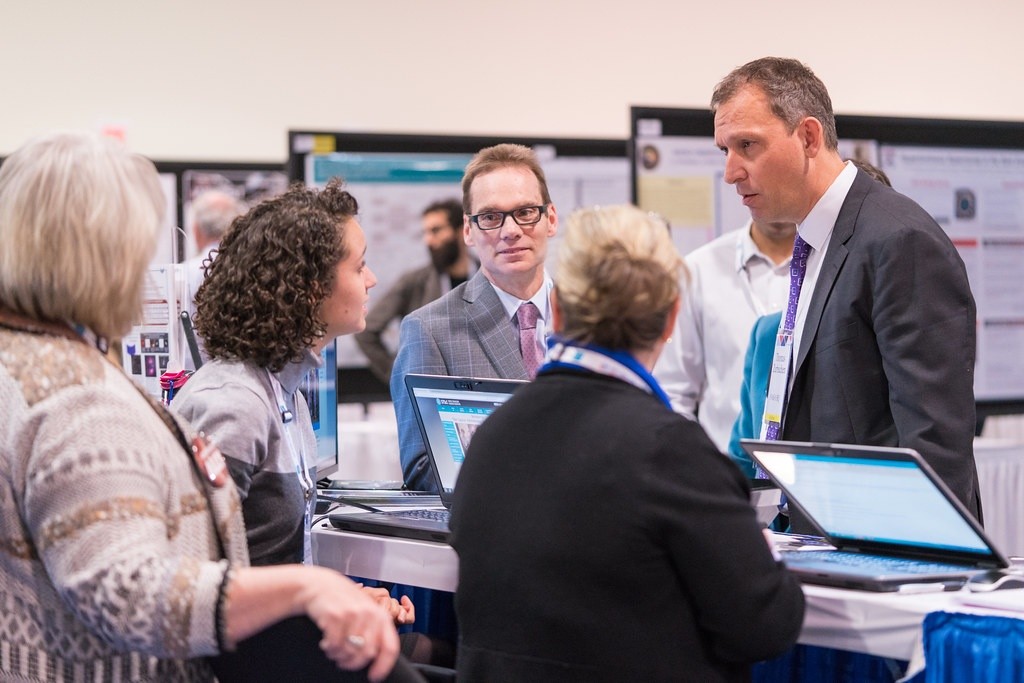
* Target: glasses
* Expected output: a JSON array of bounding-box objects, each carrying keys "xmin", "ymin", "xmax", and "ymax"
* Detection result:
[{"xmin": 462, "ymin": 202, "xmax": 548, "ymax": 232}]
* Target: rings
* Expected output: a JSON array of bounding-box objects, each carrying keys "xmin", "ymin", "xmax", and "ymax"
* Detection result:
[{"xmin": 346, "ymin": 635, "xmax": 363, "ymax": 645}]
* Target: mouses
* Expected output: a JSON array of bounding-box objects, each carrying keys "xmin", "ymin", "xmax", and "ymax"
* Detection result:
[{"xmin": 967, "ymin": 570, "xmax": 1023, "ymax": 593}]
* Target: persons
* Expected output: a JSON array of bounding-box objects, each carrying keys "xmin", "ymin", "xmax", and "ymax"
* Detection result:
[
  {"xmin": 165, "ymin": 177, "xmax": 437, "ymax": 664},
  {"xmin": 167, "ymin": 183, "xmax": 247, "ymax": 372},
  {"xmin": 711, "ymin": 57, "xmax": 985, "ymax": 533},
  {"xmin": 448, "ymin": 205, "xmax": 810, "ymax": 681},
  {"xmin": 355, "ymin": 195, "xmax": 478, "ymax": 382},
  {"xmin": 650, "ymin": 216, "xmax": 800, "ymax": 491},
  {"xmin": 0, "ymin": 135, "xmax": 400, "ymax": 683},
  {"xmin": 390, "ymin": 143, "xmax": 560, "ymax": 498}
]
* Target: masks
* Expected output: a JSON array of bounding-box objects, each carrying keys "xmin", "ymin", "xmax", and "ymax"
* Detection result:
[{"xmin": 429, "ymin": 237, "xmax": 460, "ymax": 275}]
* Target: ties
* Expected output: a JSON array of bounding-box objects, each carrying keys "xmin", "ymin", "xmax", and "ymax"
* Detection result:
[
  {"xmin": 514, "ymin": 300, "xmax": 546, "ymax": 384},
  {"xmin": 757, "ymin": 235, "xmax": 812, "ymax": 482}
]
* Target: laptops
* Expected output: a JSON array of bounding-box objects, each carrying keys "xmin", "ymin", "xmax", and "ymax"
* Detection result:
[
  {"xmin": 326, "ymin": 373, "xmax": 532, "ymax": 543},
  {"xmin": 739, "ymin": 438, "xmax": 1008, "ymax": 595}
]
